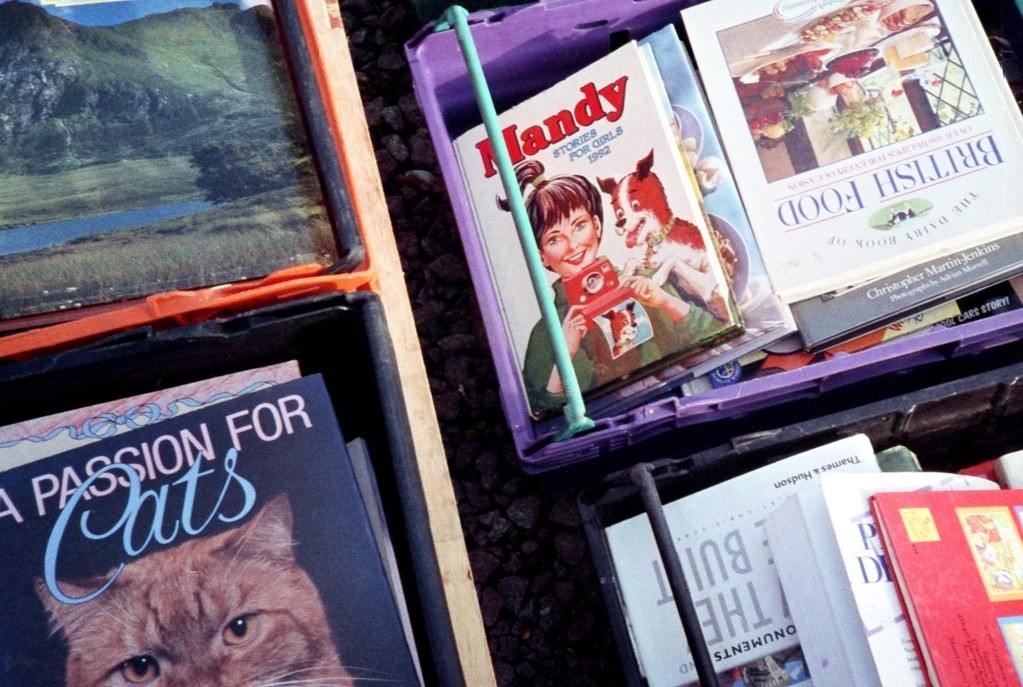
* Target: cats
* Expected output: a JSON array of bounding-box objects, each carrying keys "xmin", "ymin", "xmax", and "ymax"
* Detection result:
[{"xmin": 36, "ymin": 493, "xmax": 356, "ymax": 687}]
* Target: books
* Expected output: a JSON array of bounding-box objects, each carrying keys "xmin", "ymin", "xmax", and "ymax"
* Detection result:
[
  {"xmin": 451, "ymin": 0, "xmax": 1022, "ymax": 421},
  {"xmin": 602, "ymin": 432, "xmax": 1023, "ymax": 687},
  {"xmin": 0, "ymin": 0, "xmax": 340, "ymax": 333},
  {"xmin": 1, "ymin": 359, "xmax": 426, "ymax": 687}
]
